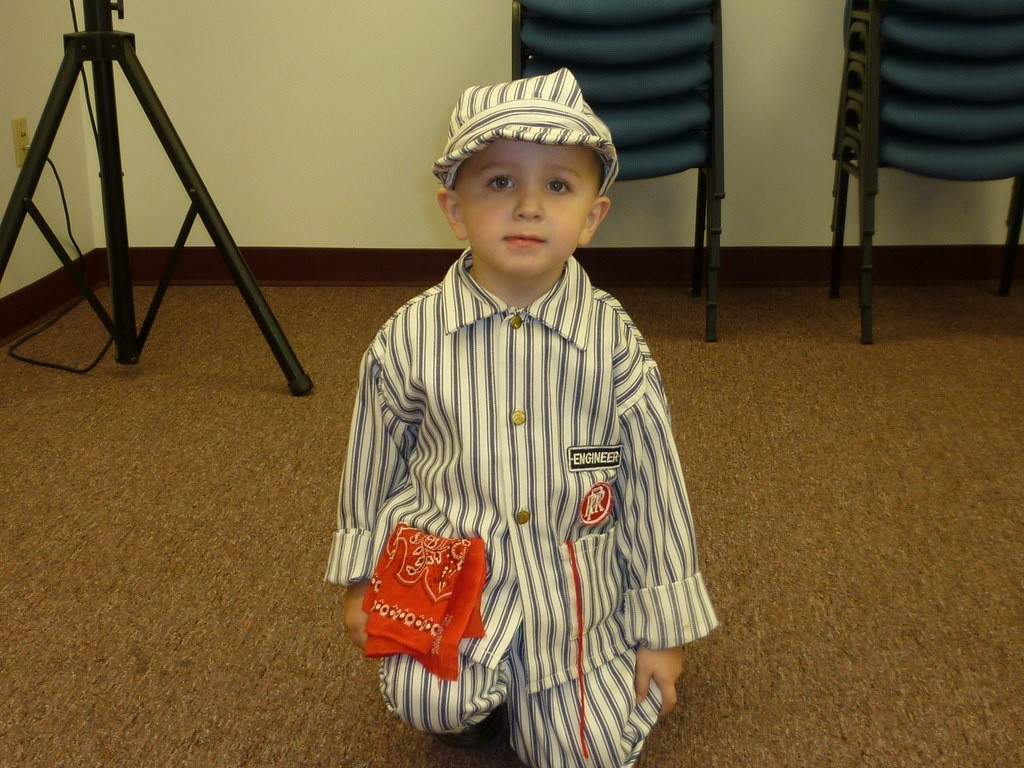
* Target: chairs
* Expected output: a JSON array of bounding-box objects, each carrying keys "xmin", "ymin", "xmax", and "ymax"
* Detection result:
[{"xmin": 515, "ymin": 1, "xmax": 1024, "ymax": 346}]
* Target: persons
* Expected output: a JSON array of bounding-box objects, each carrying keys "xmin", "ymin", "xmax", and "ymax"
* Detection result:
[{"xmin": 326, "ymin": 68, "xmax": 717, "ymax": 768}]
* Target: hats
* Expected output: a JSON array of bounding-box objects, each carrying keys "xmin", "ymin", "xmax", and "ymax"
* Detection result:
[{"xmin": 432, "ymin": 66, "xmax": 619, "ymax": 200}]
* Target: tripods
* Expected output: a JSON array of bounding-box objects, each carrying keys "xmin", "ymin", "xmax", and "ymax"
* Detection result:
[{"xmin": 0, "ymin": 0, "xmax": 314, "ymax": 397}]
